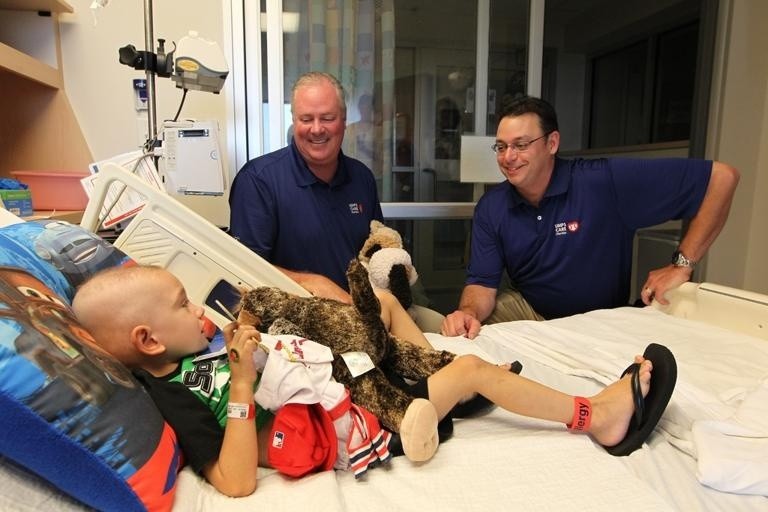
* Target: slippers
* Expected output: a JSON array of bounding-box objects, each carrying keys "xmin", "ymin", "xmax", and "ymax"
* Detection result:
[
  {"xmin": 452, "ymin": 360, "xmax": 522, "ymax": 419},
  {"xmin": 606, "ymin": 342, "xmax": 676, "ymax": 455}
]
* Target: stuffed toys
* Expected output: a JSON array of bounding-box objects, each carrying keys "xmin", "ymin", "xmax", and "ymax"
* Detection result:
[
  {"xmin": 361, "ymin": 218, "xmax": 421, "ymax": 310},
  {"xmin": 238, "ymin": 262, "xmax": 475, "ymax": 464}
]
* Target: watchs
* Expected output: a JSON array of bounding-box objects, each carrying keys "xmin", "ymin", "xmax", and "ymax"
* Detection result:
[{"xmin": 670, "ymin": 250, "xmax": 698, "ymax": 268}]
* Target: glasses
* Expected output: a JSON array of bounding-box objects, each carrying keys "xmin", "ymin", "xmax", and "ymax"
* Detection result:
[{"xmin": 492, "ymin": 132, "xmax": 548, "ymax": 154}]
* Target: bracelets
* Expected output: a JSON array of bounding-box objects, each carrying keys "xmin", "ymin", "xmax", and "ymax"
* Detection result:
[{"xmin": 225, "ymin": 402, "xmax": 256, "ymax": 421}]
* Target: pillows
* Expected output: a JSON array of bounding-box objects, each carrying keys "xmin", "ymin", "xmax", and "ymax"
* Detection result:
[{"xmin": 0, "ymin": 219, "xmax": 224, "ymax": 512}]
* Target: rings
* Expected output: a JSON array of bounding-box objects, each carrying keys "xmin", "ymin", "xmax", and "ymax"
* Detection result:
[{"xmin": 645, "ymin": 287, "xmax": 652, "ymax": 293}]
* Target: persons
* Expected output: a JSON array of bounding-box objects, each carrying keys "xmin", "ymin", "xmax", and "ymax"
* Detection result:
[
  {"xmin": 228, "ymin": 72, "xmax": 445, "ymax": 333},
  {"xmin": 440, "ymin": 95, "xmax": 740, "ymax": 340},
  {"xmin": 342, "ymin": 92, "xmax": 384, "ymax": 201},
  {"xmin": 71, "ymin": 264, "xmax": 678, "ymax": 496}
]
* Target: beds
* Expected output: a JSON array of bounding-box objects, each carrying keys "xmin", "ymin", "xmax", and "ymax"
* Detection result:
[{"xmin": 0, "ymin": 168, "xmax": 767, "ymax": 512}]
